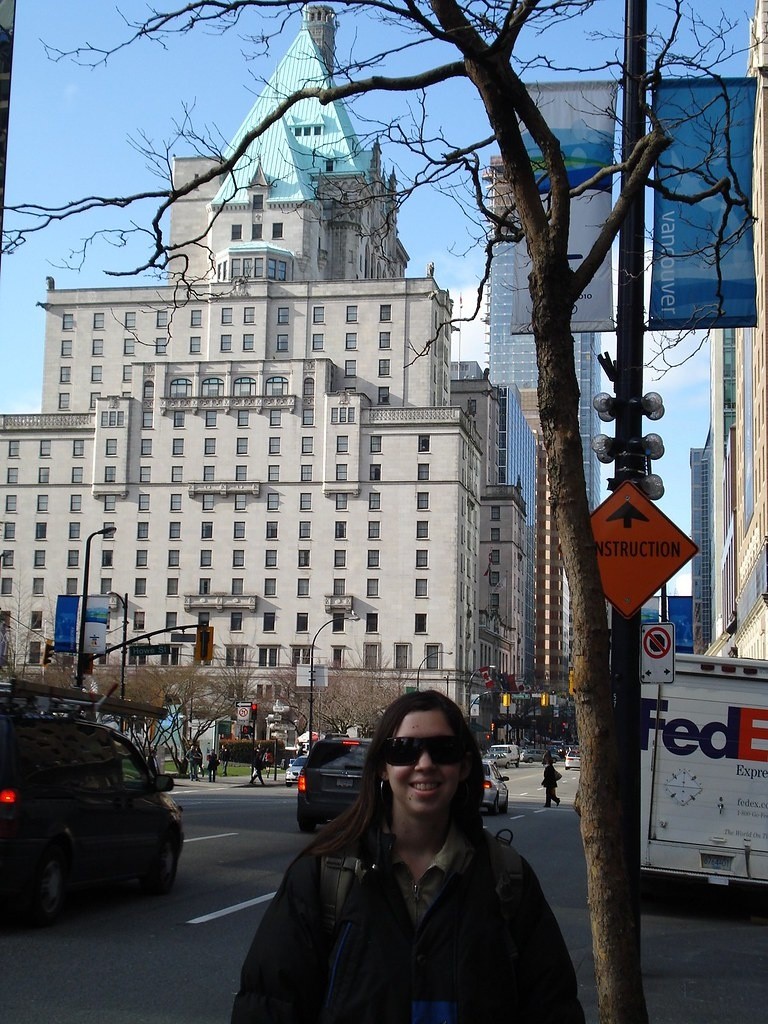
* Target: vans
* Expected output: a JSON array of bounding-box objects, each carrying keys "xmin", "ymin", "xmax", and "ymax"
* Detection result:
[{"xmin": 491, "ymin": 744, "xmax": 520, "ymax": 768}]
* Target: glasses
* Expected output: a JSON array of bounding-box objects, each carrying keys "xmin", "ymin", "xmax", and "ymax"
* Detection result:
[{"xmin": 384, "ymin": 735, "xmax": 463, "ymax": 766}]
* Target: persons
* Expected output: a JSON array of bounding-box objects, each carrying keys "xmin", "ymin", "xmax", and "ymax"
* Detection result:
[
  {"xmin": 152, "ymin": 750, "xmax": 160, "ymax": 768},
  {"xmin": 185, "ymin": 745, "xmax": 205, "ymax": 780},
  {"xmin": 231, "ymin": 689, "xmax": 586, "ymax": 1024},
  {"xmin": 542, "ymin": 759, "xmax": 560, "ymax": 807},
  {"xmin": 298, "ymin": 746, "xmax": 303, "ymax": 756},
  {"xmin": 265, "ymin": 748, "xmax": 273, "ymax": 778},
  {"xmin": 206, "ymin": 749, "xmax": 218, "ymax": 782},
  {"xmin": 219, "ymin": 744, "xmax": 230, "ymax": 776},
  {"xmin": 250, "ymin": 753, "xmax": 265, "ymax": 785}
]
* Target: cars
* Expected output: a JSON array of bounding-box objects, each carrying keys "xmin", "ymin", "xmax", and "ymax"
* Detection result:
[
  {"xmin": 482, "ymin": 752, "xmax": 510, "ymax": 769},
  {"xmin": 482, "ymin": 759, "xmax": 509, "ymax": 816},
  {"xmin": 564, "ymin": 750, "xmax": 581, "ymax": 770},
  {"xmin": 524, "ymin": 738, "xmax": 535, "ymax": 746},
  {"xmin": 519, "ymin": 748, "xmax": 561, "ymax": 764},
  {"xmin": 285, "ymin": 755, "xmax": 308, "ymax": 787}
]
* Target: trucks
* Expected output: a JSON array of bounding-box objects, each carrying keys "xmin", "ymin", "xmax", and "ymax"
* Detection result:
[{"xmin": 635, "ymin": 652, "xmax": 768, "ymax": 908}]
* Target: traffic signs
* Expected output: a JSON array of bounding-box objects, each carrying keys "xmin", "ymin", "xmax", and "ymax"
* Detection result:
[{"xmin": 558, "ymin": 478, "xmax": 701, "ymax": 620}]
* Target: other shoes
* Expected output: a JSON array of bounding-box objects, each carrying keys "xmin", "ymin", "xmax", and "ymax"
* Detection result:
[
  {"xmin": 543, "ymin": 805, "xmax": 551, "ymax": 807},
  {"xmin": 557, "ymin": 798, "xmax": 560, "ymax": 806}
]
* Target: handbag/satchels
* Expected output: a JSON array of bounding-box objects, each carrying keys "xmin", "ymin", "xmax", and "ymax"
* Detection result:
[{"xmin": 554, "ymin": 770, "xmax": 562, "ymax": 781}]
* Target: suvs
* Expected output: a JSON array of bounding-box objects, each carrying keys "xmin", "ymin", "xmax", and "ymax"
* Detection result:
[
  {"xmin": 296, "ymin": 733, "xmax": 374, "ymax": 835},
  {"xmin": 1, "ymin": 707, "xmax": 184, "ymax": 929}
]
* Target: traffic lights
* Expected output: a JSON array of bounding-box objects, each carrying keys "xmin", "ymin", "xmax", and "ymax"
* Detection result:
[
  {"xmin": 251, "ymin": 704, "xmax": 258, "ymax": 720},
  {"xmin": 242, "ymin": 726, "xmax": 253, "ymax": 734},
  {"xmin": 42, "ymin": 644, "xmax": 55, "ymax": 665}
]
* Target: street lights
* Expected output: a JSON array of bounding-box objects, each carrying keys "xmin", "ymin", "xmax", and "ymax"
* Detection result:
[
  {"xmin": 106, "ymin": 591, "xmax": 128, "ymax": 733},
  {"xmin": 308, "ymin": 616, "xmax": 361, "ymax": 748},
  {"xmin": 416, "ymin": 651, "xmax": 453, "ymax": 691},
  {"xmin": 77, "ymin": 525, "xmax": 118, "ymax": 689},
  {"xmin": 466, "ymin": 665, "xmax": 497, "ymax": 724}
]
